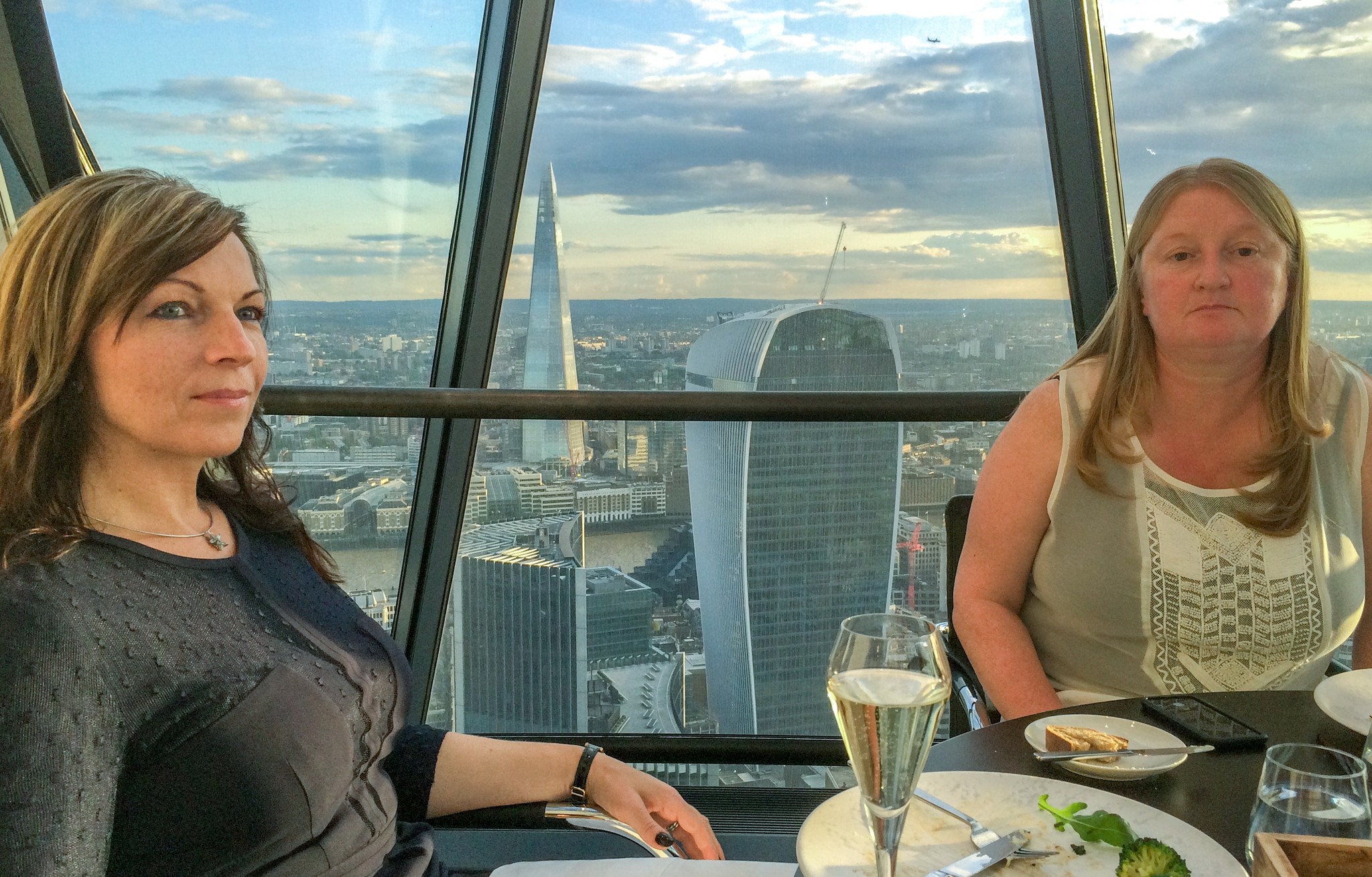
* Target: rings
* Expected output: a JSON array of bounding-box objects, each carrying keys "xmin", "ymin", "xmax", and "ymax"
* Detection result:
[{"xmin": 667, "ymin": 822, "xmax": 679, "ymax": 832}]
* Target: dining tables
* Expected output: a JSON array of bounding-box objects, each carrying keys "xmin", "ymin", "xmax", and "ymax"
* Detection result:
[{"xmin": 927, "ymin": 692, "xmax": 1372, "ymax": 877}]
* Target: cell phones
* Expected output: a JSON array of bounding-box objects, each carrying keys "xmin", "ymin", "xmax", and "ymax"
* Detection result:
[{"xmin": 1140, "ymin": 693, "xmax": 1269, "ymax": 750}]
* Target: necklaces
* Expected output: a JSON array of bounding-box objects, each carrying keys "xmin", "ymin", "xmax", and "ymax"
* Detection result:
[{"xmin": 81, "ymin": 496, "xmax": 229, "ymax": 552}]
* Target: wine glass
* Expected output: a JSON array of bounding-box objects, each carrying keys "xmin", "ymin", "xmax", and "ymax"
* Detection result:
[{"xmin": 824, "ymin": 613, "xmax": 952, "ymax": 877}]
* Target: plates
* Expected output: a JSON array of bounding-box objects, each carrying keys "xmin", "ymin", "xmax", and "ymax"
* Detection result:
[
  {"xmin": 1024, "ymin": 715, "xmax": 1188, "ymax": 781},
  {"xmin": 1313, "ymin": 668, "xmax": 1372, "ymax": 736},
  {"xmin": 795, "ymin": 771, "xmax": 1252, "ymax": 877}
]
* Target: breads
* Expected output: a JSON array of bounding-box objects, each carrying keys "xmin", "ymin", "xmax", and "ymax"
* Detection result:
[{"xmin": 1046, "ymin": 725, "xmax": 1129, "ymax": 763}]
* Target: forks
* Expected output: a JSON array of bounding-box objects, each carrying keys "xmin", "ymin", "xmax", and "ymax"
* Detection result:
[{"xmin": 847, "ymin": 760, "xmax": 1060, "ymax": 861}]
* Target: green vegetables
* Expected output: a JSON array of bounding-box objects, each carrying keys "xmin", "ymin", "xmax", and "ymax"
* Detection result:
[{"xmin": 1038, "ymin": 794, "xmax": 1134, "ymax": 848}]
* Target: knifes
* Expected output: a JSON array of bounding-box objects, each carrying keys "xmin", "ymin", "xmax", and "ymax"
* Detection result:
[
  {"xmin": 1033, "ymin": 745, "xmax": 1214, "ymax": 761},
  {"xmin": 921, "ymin": 829, "xmax": 1032, "ymax": 877}
]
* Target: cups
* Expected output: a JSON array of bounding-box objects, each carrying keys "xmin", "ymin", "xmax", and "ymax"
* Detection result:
[
  {"xmin": 1361, "ymin": 722, "xmax": 1372, "ymax": 800},
  {"xmin": 1245, "ymin": 744, "xmax": 1372, "ymax": 869}
]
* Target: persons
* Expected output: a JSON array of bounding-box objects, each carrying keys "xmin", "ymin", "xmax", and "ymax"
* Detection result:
[
  {"xmin": 953, "ymin": 157, "xmax": 1372, "ymax": 723},
  {"xmin": 1, "ymin": 167, "xmax": 724, "ymax": 877}
]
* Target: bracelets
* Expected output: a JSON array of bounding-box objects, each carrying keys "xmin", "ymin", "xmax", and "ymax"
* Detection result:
[{"xmin": 570, "ymin": 742, "xmax": 604, "ymax": 803}]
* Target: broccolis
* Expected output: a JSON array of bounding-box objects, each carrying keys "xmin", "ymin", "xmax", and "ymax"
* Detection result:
[{"xmin": 1116, "ymin": 837, "xmax": 1192, "ymax": 877}]
